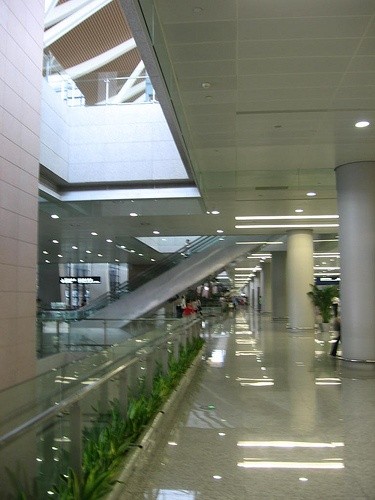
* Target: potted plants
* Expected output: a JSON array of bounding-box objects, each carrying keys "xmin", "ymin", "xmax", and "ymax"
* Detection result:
[{"xmin": 306, "ymin": 283, "xmax": 339, "ymax": 333}]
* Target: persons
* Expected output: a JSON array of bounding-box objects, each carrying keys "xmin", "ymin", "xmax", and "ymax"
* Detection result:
[
  {"xmin": 328, "ymin": 296, "xmax": 340, "ymax": 358},
  {"xmin": 175, "ymin": 293, "xmax": 248, "ymax": 319}
]
